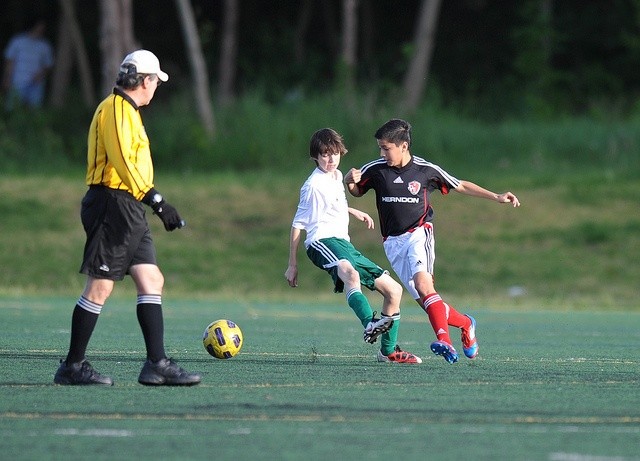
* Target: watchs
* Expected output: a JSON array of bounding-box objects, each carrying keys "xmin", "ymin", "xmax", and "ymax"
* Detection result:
[{"xmin": 151, "ymin": 193, "xmax": 164, "ymax": 209}]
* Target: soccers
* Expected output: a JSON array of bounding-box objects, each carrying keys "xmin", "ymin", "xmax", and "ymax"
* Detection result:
[{"xmin": 202, "ymin": 319, "xmax": 243, "ymax": 359}]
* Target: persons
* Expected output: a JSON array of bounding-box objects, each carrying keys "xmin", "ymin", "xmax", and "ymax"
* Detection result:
[
  {"xmin": 3, "ymin": 20, "xmax": 55, "ymax": 111},
  {"xmin": 284, "ymin": 128, "xmax": 422, "ymax": 363},
  {"xmin": 345, "ymin": 119, "xmax": 520, "ymax": 363},
  {"xmin": 54, "ymin": 49, "xmax": 201, "ymax": 386}
]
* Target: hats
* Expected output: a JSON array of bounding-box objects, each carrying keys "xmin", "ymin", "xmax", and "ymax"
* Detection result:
[{"xmin": 118, "ymin": 48, "xmax": 170, "ymax": 83}]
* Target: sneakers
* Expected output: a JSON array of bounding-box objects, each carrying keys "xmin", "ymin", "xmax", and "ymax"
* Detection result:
[
  {"xmin": 377, "ymin": 344, "xmax": 423, "ymax": 364},
  {"xmin": 460, "ymin": 313, "xmax": 479, "ymax": 358},
  {"xmin": 138, "ymin": 355, "xmax": 201, "ymax": 386},
  {"xmin": 54, "ymin": 356, "xmax": 115, "ymax": 385},
  {"xmin": 363, "ymin": 309, "xmax": 394, "ymax": 344},
  {"xmin": 430, "ymin": 339, "xmax": 459, "ymax": 364}
]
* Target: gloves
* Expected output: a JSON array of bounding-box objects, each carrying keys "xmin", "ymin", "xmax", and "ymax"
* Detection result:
[{"xmin": 141, "ymin": 186, "xmax": 183, "ymax": 231}]
